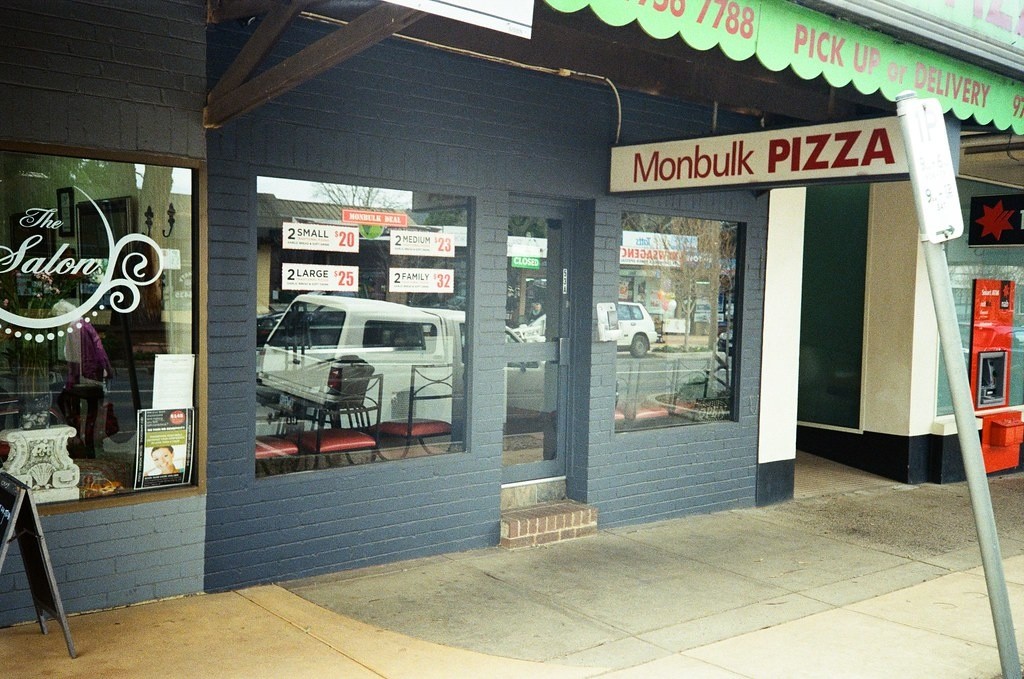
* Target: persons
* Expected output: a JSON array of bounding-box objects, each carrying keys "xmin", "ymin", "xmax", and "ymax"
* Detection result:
[
  {"xmin": 48, "ymin": 288, "xmax": 107, "ymax": 459},
  {"xmin": 144, "ymin": 446, "xmax": 184, "ymax": 476}
]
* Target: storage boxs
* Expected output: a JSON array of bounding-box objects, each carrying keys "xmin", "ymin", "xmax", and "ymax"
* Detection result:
[{"xmin": 991, "ymin": 420, "xmax": 1024, "ymax": 447}]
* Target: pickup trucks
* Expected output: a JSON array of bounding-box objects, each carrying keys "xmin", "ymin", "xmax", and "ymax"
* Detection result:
[{"xmin": 255, "ymin": 294, "xmax": 544, "ymax": 426}]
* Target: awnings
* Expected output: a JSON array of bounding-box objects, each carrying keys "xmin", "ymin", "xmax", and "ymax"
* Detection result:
[{"xmin": 543, "ymin": 0, "xmax": 1024, "ymax": 136}]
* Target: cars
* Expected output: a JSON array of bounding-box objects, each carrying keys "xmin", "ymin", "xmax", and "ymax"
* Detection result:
[
  {"xmin": 257, "ymin": 291, "xmax": 421, "ymax": 350},
  {"xmin": 527, "ymin": 301, "xmax": 659, "ymax": 357},
  {"xmin": 719, "ymin": 327, "xmax": 734, "ymax": 355},
  {"xmin": 0, "ymin": 367, "xmax": 106, "ymax": 457}
]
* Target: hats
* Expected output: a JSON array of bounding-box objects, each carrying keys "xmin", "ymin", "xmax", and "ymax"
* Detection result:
[{"xmin": 53, "ymin": 301, "xmax": 77, "ymax": 317}]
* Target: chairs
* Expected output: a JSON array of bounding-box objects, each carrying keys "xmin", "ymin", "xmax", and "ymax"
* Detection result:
[
  {"xmin": 255, "ymin": 364, "xmax": 305, "ymax": 478},
  {"xmin": 666, "ymin": 356, "xmax": 710, "ymax": 423},
  {"xmin": 619, "ymin": 359, "xmax": 676, "ymax": 428},
  {"xmin": 285, "ymin": 373, "xmax": 382, "ymax": 470},
  {"xmin": 614, "ymin": 361, "xmax": 632, "ymax": 430},
  {"xmin": 365, "ymin": 363, "xmax": 453, "ymax": 461}
]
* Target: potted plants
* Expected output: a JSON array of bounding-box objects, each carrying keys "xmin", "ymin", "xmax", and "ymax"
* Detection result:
[{"xmin": 0, "ymin": 260, "xmax": 97, "ymax": 429}]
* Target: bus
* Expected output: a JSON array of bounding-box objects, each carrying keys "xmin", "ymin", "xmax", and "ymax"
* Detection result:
[{"xmin": 322, "ymin": 225, "xmax": 738, "ymax": 424}]
